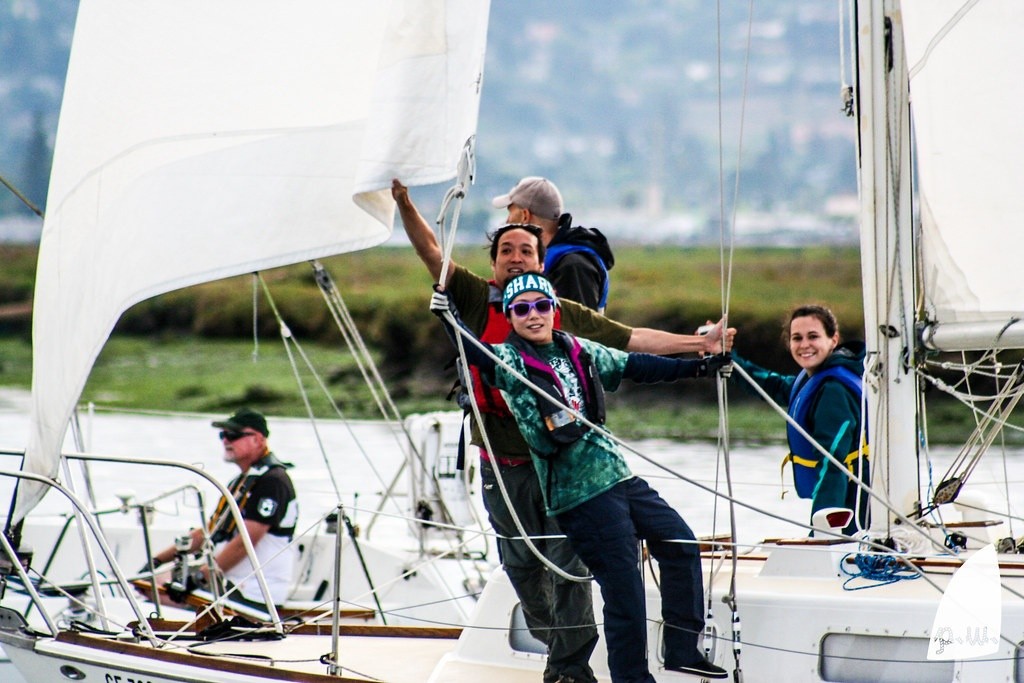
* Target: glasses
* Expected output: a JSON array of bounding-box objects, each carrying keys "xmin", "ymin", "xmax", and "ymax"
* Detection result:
[
  {"xmin": 219, "ymin": 430, "xmax": 256, "ymax": 442},
  {"xmin": 493, "ymin": 224, "xmax": 542, "ymax": 233},
  {"xmin": 508, "ymin": 298, "xmax": 555, "ymax": 316}
]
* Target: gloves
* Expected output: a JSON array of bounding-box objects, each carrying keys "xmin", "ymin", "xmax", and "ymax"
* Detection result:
[{"xmin": 698, "ymin": 351, "xmax": 733, "ymax": 378}]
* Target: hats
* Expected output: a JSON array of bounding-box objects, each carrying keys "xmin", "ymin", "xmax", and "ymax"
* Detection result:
[
  {"xmin": 492, "ymin": 177, "xmax": 564, "ymax": 221},
  {"xmin": 212, "ymin": 407, "xmax": 270, "ymax": 437}
]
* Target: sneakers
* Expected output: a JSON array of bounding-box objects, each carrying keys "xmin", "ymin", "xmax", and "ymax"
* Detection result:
[{"xmin": 664, "ymin": 659, "xmax": 729, "ymax": 679}]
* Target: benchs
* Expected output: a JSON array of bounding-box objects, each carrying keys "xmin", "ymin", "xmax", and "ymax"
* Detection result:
[{"xmin": 126, "ymin": 598, "xmax": 463, "ymax": 640}]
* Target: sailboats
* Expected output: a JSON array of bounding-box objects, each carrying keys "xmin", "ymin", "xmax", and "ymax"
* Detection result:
[{"xmin": 1, "ymin": 0, "xmax": 1022, "ymax": 682}]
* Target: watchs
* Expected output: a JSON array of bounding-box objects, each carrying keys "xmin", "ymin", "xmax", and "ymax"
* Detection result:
[{"xmin": 194, "ymin": 570, "xmax": 208, "ymax": 584}]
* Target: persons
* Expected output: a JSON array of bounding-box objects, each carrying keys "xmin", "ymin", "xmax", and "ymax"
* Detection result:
[
  {"xmin": 137, "ymin": 412, "xmax": 298, "ymax": 620},
  {"xmin": 391, "ymin": 176, "xmax": 738, "ymax": 683},
  {"xmin": 694, "ymin": 305, "xmax": 872, "ymax": 538}
]
